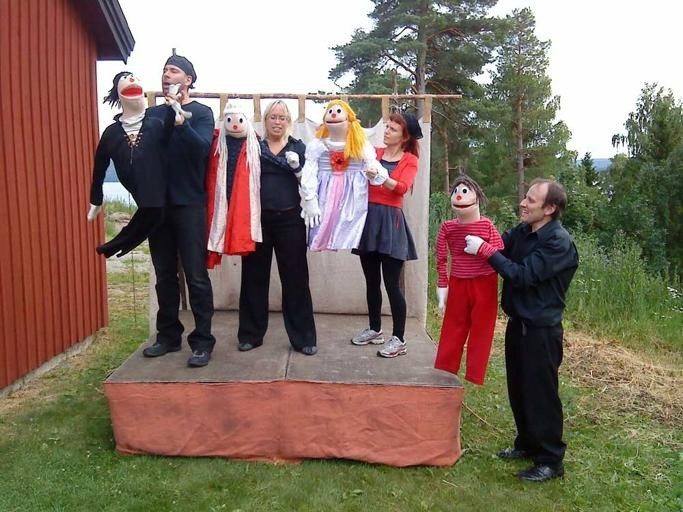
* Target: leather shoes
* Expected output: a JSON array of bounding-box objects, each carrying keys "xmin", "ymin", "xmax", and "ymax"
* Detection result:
[
  {"xmin": 496, "ymin": 449, "xmax": 532, "ymax": 459},
  {"xmin": 519, "ymin": 465, "xmax": 564, "ymax": 481}
]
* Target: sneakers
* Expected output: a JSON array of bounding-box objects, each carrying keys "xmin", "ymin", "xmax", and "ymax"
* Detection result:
[
  {"xmin": 143, "ymin": 341, "xmax": 181, "ymax": 357},
  {"xmin": 188, "ymin": 343, "xmax": 214, "ymax": 367},
  {"xmin": 351, "ymin": 328, "xmax": 385, "ymax": 345},
  {"xmin": 239, "ymin": 340, "xmax": 263, "ymax": 351},
  {"xmin": 302, "ymin": 346, "xmax": 317, "ymax": 355},
  {"xmin": 377, "ymin": 336, "xmax": 407, "ymax": 358}
]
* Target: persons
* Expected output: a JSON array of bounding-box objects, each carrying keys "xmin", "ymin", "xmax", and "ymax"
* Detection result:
[
  {"xmin": 203, "ymin": 97, "xmax": 264, "ymax": 271},
  {"xmin": 299, "ymin": 98, "xmax": 388, "ymax": 256},
  {"xmin": 464, "ymin": 177, "xmax": 580, "ymax": 481},
  {"xmin": 141, "ymin": 53, "xmax": 218, "ymax": 368},
  {"xmin": 435, "ymin": 173, "xmax": 505, "ymax": 386},
  {"xmin": 236, "ymin": 96, "xmax": 320, "ymax": 356},
  {"xmin": 352, "ymin": 111, "xmax": 426, "ymax": 358},
  {"xmin": 86, "ymin": 69, "xmax": 193, "ymax": 261}
]
